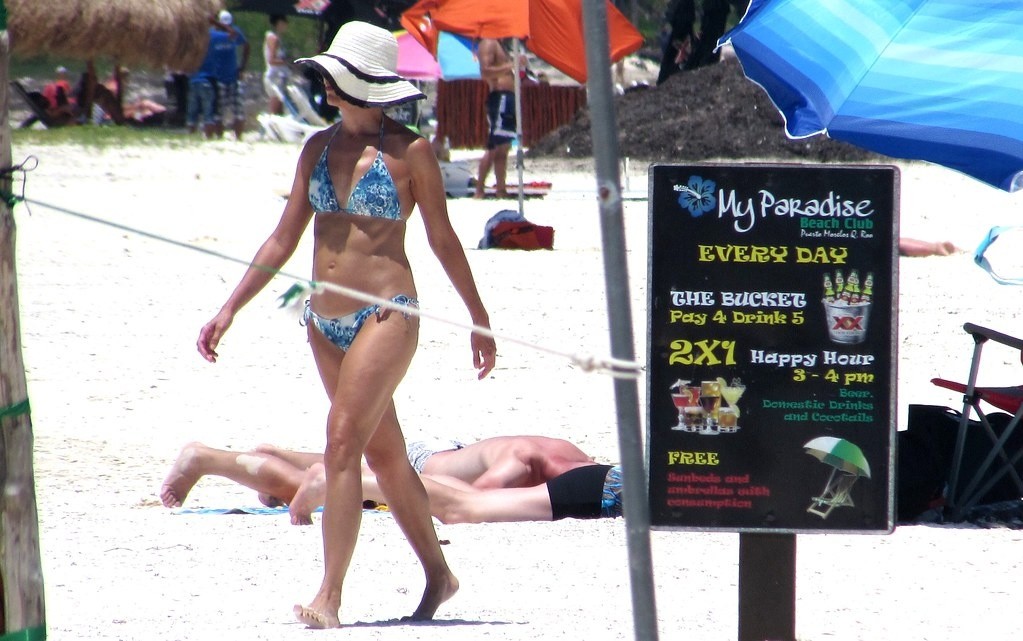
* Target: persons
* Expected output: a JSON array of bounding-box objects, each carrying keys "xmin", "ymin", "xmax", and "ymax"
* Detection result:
[
  {"xmin": 288, "ymin": 462, "xmax": 623, "ymax": 525},
  {"xmin": 263, "ymin": 16, "xmax": 289, "ymax": 114},
  {"xmin": 899, "ymin": 237, "xmax": 964, "ymax": 258},
  {"xmin": 474, "ymin": 37, "xmax": 515, "ymax": 199},
  {"xmin": 187, "ymin": 10, "xmax": 250, "ymax": 140},
  {"xmin": 43, "ymin": 65, "xmax": 166, "ymax": 126},
  {"xmin": 196, "ymin": 19, "xmax": 496, "ymax": 632},
  {"xmin": 161, "ymin": 435, "xmax": 598, "ymax": 507}
]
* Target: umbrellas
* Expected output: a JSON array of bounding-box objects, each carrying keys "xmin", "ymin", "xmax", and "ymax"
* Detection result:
[
  {"xmin": 713, "ymin": 0, "xmax": 1023, "ymax": 192},
  {"xmin": 398, "ymin": 0, "xmax": 644, "ymax": 84},
  {"xmin": 803, "ymin": 436, "xmax": 870, "ymax": 499},
  {"xmin": 391, "ymin": 28, "xmax": 482, "ymax": 82}
]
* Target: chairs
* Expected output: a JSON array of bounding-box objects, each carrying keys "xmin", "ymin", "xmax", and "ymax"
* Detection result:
[
  {"xmin": 252, "ymin": 80, "xmax": 333, "ymax": 147},
  {"xmin": 927, "ymin": 321, "xmax": 1023, "ymax": 524},
  {"xmin": 8, "ymin": 76, "xmax": 149, "ymax": 130}
]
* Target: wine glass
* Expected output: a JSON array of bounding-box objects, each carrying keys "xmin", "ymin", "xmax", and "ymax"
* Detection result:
[
  {"xmin": 721, "ymin": 386, "xmax": 746, "ymax": 430},
  {"xmin": 671, "ymin": 394, "xmax": 690, "ymax": 431},
  {"xmin": 699, "ymin": 396, "xmax": 720, "ymax": 436}
]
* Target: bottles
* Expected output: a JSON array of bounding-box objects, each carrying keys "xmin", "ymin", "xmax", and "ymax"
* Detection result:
[
  {"xmin": 823, "ymin": 272, "xmax": 835, "ymax": 301},
  {"xmin": 836, "ymin": 269, "xmax": 845, "ymax": 299},
  {"xmin": 862, "ymin": 272, "xmax": 873, "ymax": 302},
  {"xmin": 850, "ymin": 279, "xmax": 860, "ymax": 303},
  {"xmin": 841, "ymin": 270, "xmax": 858, "ymax": 304}
]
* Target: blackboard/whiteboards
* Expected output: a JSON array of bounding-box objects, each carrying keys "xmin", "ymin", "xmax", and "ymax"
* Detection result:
[{"xmin": 645, "ymin": 162, "xmax": 899, "ymax": 536}]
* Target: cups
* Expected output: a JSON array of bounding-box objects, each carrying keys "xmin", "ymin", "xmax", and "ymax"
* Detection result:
[
  {"xmin": 679, "ymin": 386, "xmax": 701, "ymax": 432},
  {"xmin": 718, "ymin": 408, "xmax": 737, "ymax": 433},
  {"xmin": 703, "ymin": 382, "xmax": 721, "ymax": 419}
]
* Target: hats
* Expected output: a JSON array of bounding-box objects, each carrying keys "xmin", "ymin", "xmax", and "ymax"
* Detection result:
[
  {"xmin": 218, "ymin": 9, "xmax": 233, "ymax": 25},
  {"xmin": 293, "ymin": 20, "xmax": 428, "ymax": 109}
]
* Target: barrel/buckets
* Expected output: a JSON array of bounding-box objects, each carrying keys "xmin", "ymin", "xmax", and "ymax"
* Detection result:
[{"xmin": 821, "ymin": 298, "xmax": 871, "ymax": 344}]
